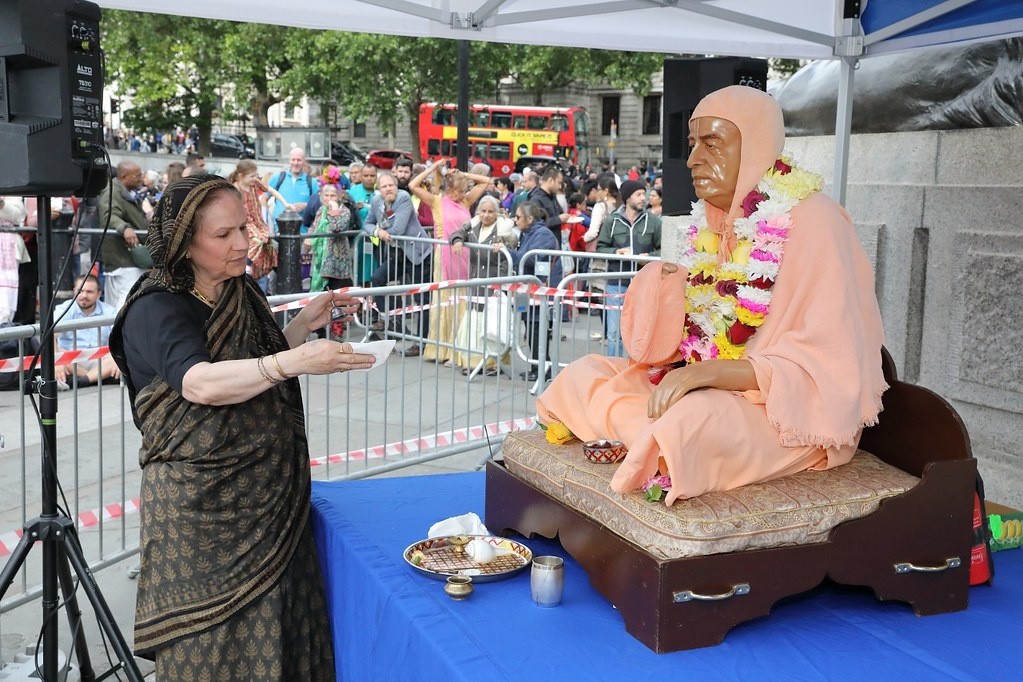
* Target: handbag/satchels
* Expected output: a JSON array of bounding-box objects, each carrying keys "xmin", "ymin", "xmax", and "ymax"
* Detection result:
[{"xmin": 585, "ymin": 201, "xmax": 609, "ymax": 252}]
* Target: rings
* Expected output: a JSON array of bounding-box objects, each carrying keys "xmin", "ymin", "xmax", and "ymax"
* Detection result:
[{"xmin": 339, "ymin": 344, "xmax": 344, "ymax": 353}]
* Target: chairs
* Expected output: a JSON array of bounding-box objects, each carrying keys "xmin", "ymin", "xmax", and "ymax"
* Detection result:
[{"xmin": 485, "ymin": 345, "xmax": 979, "ymax": 653}]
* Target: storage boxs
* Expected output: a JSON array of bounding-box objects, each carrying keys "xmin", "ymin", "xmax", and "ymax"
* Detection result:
[{"xmin": 985, "ymin": 499, "xmax": 1023, "ymax": 555}]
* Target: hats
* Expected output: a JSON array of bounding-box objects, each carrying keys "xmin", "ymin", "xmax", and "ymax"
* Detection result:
[{"xmin": 620, "ymin": 180, "xmax": 647, "ymax": 201}]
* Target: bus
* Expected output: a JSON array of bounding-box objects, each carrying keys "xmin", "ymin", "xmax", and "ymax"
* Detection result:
[{"xmin": 418, "ymin": 100, "xmax": 584, "ymax": 179}]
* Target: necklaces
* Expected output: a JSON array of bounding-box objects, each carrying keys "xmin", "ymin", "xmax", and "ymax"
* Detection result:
[{"xmin": 193, "ymin": 287, "xmax": 218, "ymax": 309}]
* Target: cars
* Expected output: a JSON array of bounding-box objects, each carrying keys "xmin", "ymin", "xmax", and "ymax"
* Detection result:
[
  {"xmin": 364, "ymin": 150, "xmax": 416, "ymax": 174},
  {"xmin": 189, "ymin": 132, "xmax": 253, "ymax": 158},
  {"xmin": 295, "ymin": 132, "xmax": 365, "ymax": 169}
]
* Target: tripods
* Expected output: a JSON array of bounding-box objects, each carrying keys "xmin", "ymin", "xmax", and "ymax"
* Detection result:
[{"xmin": 0, "ymin": 196, "xmax": 147, "ymax": 682}]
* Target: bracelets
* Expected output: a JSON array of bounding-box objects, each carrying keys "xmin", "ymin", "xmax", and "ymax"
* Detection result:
[{"xmin": 257, "ymin": 354, "xmax": 288, "ymax": 385}]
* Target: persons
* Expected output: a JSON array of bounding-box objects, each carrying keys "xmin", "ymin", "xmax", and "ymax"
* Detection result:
[
  {"xmin": 109, "ymin": 173, "xmax": 333, "ymax": 682},
  {"xmin": 103, "ymin": 123, "xmax": 199, "ymax": 155},
  {"xmin": 0, "ymin": 153, "xmax": 663, "ymax": 391},
  {"xmin": 537, "ymin": 85, "xmax": 888, "ymax": 497}
]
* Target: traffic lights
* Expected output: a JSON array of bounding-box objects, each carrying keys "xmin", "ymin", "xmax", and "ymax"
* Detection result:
[{"xmin": 111, "ymin": 97, "xmax": 119, "ymax": 114}]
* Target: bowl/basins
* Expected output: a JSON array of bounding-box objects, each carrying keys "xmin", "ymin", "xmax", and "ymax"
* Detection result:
[{"xmin": 581, "ymin": 439, "xmax": 623, "ymax": 464}]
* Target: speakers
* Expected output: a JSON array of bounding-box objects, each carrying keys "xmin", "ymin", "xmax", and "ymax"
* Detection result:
[{"xmin": 0, "ymin": 0, "xmax": 108, "ymax": 199}]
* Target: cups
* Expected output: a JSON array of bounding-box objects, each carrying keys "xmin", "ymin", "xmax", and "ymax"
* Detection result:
[{"xmin": 531, "ymin": 554, "xmax": 565, "ymax": 607}]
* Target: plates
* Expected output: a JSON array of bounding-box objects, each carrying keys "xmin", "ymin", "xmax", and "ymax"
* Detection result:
[{"xmin": 565, "ymin": 217, "xmax": 585, "ymax": 223}]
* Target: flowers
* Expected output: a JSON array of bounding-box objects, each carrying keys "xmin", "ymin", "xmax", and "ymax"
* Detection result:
[
  {"xmin": 642, "ymin": 474, "xmax": 671, "ymax": 502},
  {"xmin": 534, "ymin": 417, "xmax": 575, "ymax": 446}
]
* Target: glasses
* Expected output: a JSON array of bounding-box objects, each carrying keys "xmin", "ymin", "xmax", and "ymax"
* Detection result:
[{"xmin": 516, "ymin": 215, "xmax": 531, "ymax": 220}]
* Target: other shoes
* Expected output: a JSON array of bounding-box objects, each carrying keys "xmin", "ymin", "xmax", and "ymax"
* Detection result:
[
  {"xmin": 519, "ymin": 371, "xmax": 551, "ymax": 381},
  {"xmin": 462, "ymin": 367, "xmax": 504, "ymax": 376},
  {"xmin": 443, "ymin": 359, "xmax": 457, "ymax": 367},
  {"xmin": 26, "ymin": 376, "xmax": 70, "ymax": 391},
  {"xmin": 400, "ymin": 343, "xmax": 424, "ymax": 357}
]
